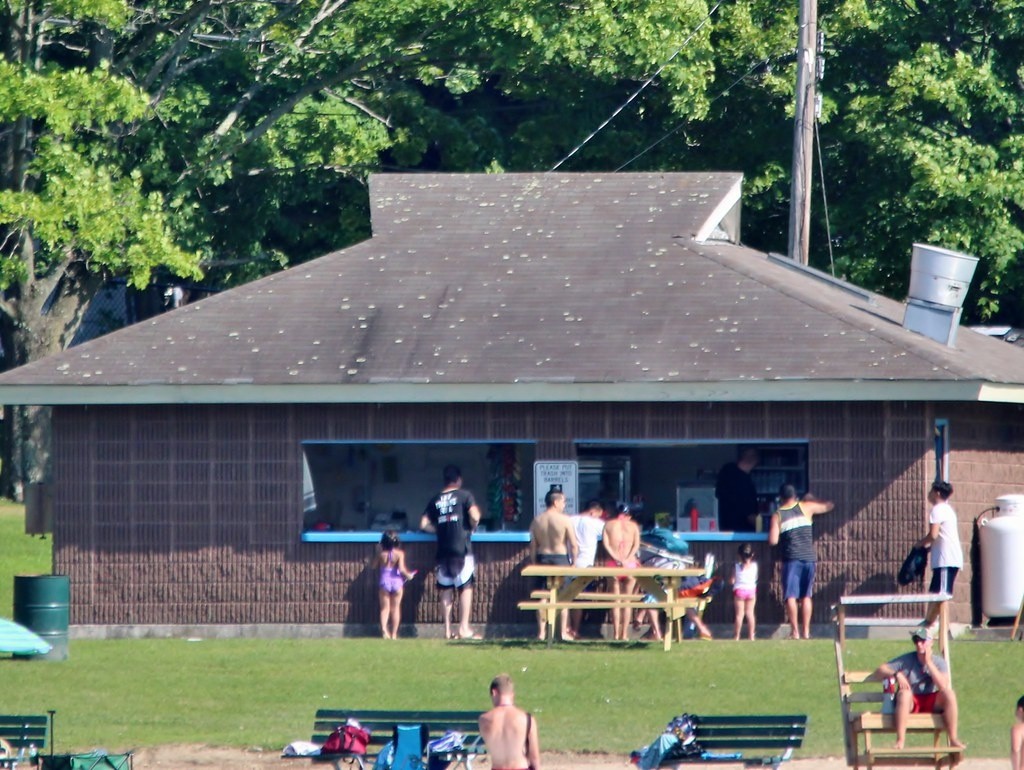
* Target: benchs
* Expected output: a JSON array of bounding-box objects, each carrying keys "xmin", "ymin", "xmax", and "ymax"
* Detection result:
[
  {"xmin": 631, "ymin": 713, "xmax": 809, "ymax": 770},
  {"xmin": 281, "ymin": 708, "xmax": 491, "ymax": 770},
  {"xmin": 0, "ymin": 715, "xmax": 48, "ymax": 770},
  {"xmin": 517, "ymin": 589, "xmax": 714, "ymax": 611}
]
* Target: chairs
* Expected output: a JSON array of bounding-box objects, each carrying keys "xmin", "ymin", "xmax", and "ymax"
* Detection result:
[
  {"xmin": 831, "ymin": 591, "xmax": 964, "ymax": 770},
  {"xmin": 373, "ymin": 723, "xmax": 432, "ymax": 770}
]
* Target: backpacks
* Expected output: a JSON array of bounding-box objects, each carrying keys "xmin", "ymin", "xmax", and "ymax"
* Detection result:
[{"xmin": 639, "ymin": 527, "xmax": 688, "ymax": 555}]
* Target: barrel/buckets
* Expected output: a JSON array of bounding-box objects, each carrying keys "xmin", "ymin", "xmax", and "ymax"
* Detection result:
[{"xmin": 13, "ymin": 575, "xmax": 72, "ymax": 663}]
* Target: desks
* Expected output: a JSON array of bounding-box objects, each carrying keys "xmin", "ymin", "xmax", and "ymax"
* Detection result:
[{"xmin": 522, "ymin": 565, "xmax": 705, "ymax": 652}]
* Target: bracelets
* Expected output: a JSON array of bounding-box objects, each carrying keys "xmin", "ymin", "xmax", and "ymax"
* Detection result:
[{"xmin": 894, "ymin": 670, "xmax": 899, "ymax": 676}]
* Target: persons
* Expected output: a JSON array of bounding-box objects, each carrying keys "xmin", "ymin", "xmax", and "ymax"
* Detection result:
[
  {"xmin": 715, "ymin": 447, "xmax": 762, "ymax": 532},
  {"xmin": 635, "ymin": 543, "xmax": 712, "ymax": 640},
  {"xmin": 728, "ymin": 542, "xmax": 759, "ymax": 641},
  {"xmin": 874, "ymin": 627, "xmax": 966, "ymax": 749},
  {"xmin": 369, "ymin": 531, "xmax": 414, "ymax": 640},
  {"xmin": 418, "ymin": 465, "xmax": 484, "ymax": 640},
  {"xmin": 769, "ymin": 484, "xmax": 836, "ymax": 640},
  {"xmin": 528, "ymin": 490, "xmax": 577, "ymax": 641},
  {"xmin": 566, "ymin": 499, "xmax": 607, "ymax": 633},
  {"xmin": 478, "ymin": 674, "xmax": 541, "ymax": 770},
  {"xmin": 601, "ymin": 503, "xmax": 640, "ymax": 640},
  {"xmin": 1010, "ymin": 696, "xmax": 1024, "ymax": 770},
  {"xmin": 913, "ymin": 480, "xmax": 963, "ymax": 638}
]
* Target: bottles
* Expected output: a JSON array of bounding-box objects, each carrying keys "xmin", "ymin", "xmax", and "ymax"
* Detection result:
[{"xmin": 690, "ymin": 507, "xmax": 699, "ymax": 532}]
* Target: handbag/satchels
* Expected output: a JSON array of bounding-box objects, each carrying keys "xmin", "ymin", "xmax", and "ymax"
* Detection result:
[
  {"xmin": 428, "ymin": 728, "xmax": 463, "ymax": 770},
  {"xmin": 882, "ymin": 676, "xmax": 895, "ymax": 715},
  {"xmin": 322, "ymin": 718, "xmax": 372, "ymax": 765},
  {"xmin": 898, "ymin": 545, "xmax": 929, "ymax": 588},
  {"xmin": 283, "ymin": 740, "xmax": 324, "ymax": 756}
]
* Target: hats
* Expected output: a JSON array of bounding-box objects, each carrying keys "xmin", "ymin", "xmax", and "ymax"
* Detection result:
[
  {"xmin": 912, "ymin": 628, "xmax": 934, "ymax": 639},
  {"xmin": 619, "ymin": 504, "xmax": 634, "ymax": 516}
]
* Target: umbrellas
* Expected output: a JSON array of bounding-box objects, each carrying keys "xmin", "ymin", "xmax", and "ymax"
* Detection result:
[{"xmin": 0, "ymin": 618, "xmax": 52, "ymax": 655}]
